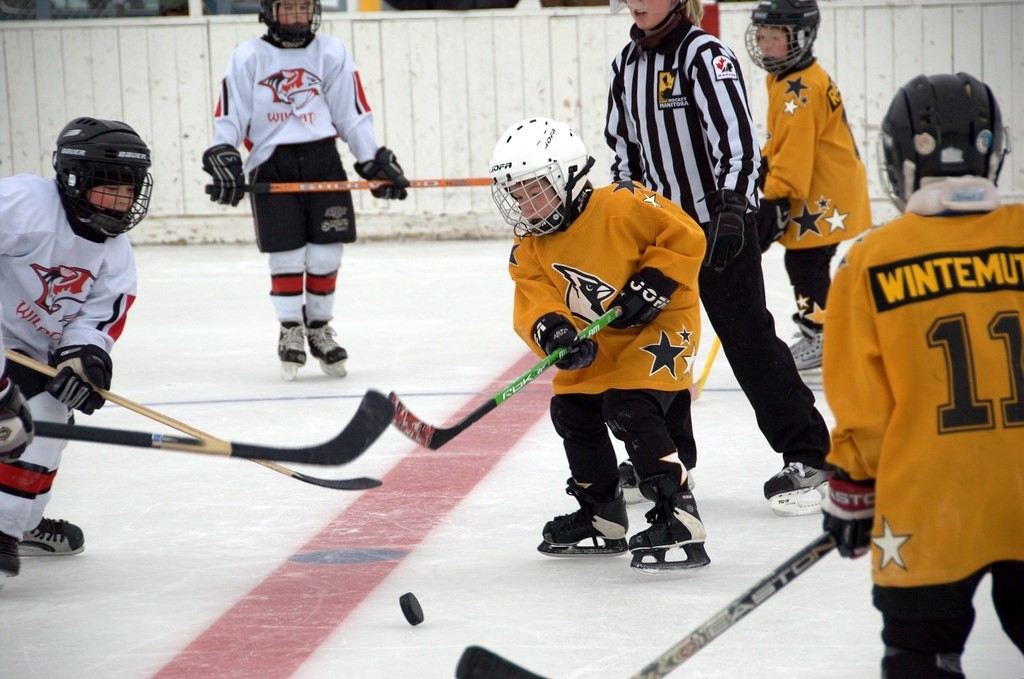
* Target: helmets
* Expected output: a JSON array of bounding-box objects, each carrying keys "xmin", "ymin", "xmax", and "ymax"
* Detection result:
[
  {"xmin": 878, "ymin": 71, "xmax": 1012, "ymax": 211},
  {"xmin": 489, "ymin": 117, "xmax": 594, "ymax": 236},
  {"xmin": 750, "ymin": 0, "xmax": 821, "ymax": 70},
  {"xmin": 259, "ymin": 1, "xmax": 321, "ymax": 41},
  {"xmin": 51, "ymin": 116, "xmax": 152, "ymax": 243}
]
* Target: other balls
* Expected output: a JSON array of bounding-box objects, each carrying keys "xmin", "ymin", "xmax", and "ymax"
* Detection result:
[{"xmin": 398, "ymin": 591, "xmax": 426, "ymax": 626}]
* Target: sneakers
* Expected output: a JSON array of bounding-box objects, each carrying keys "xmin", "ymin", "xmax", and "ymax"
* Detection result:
[
  {"xmin": 628, "ymin": 489, "xmax": 711, "ymax": 573},
  {"xmin": 536, "ymin": 478, "xmax": 630, "ymax": 558},
  {"xmin": 787, "ymin": 328, "xmax": 829, "ymax": 370},
  {"xmin": 277, "ymin": 320, "xmax": 308, "ymax": 378},
  {"xmin": 0, "ymin": 532, "xmax": 21, "ymax": 577},
  {"xmin": 619, "ymin": 458, "xmax": 696, "ymax": 505},
  {"xmin": 19, "ymin": 517, "xmax": 85, "ymax": 557},
  {"xmin": 301, "ymin": 304, "xmax": 348, "ymax": 377},
  {"xmin": 763, "ymin": 462, "xmax": 829, "ymax": 517}
]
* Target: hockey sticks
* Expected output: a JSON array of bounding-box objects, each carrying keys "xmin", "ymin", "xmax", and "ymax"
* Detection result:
[
  {"xmin": 30, "ymin": 388, "xmax": 398, "ymax": 469},
  {"xmin": 386, "ymin": 304, "xmax": 626, "ymax": 451},
  {"xmin": 1, "ymin": 343, "xmax": 384, "ymax": 491},
  {"xmin": 692, "ymin": 336, "xmax": 722, "ymax": 402},
  {"xmin": 204, "ymin": 177, "xmax": 495, "ymax": 194},
  {"xmin": 453, "ymin": 530, "xmax": 840, "ymax": 679}
]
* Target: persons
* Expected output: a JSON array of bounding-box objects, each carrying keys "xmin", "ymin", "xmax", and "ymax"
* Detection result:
[
  {"xmin": 488, "ymin": 116, "xmax": 709, "ymax": 553},
  {"xmin": 821, "ymin": 72, "xmax": 1024, "ymax": 679},
  {"xmin": 743, "ymin": 0, "xmax": 873, "ymax": 373},
  {"xmin": 200, "ymin": 0, "xmax": 410, "ymax": 366},
  {"xmin": 601, "ymin": 0, "xmax": 840, "ymax": 501},
  {"xmin": 0, "ymin": 114, "xmax": 155, "ymax": 579}
]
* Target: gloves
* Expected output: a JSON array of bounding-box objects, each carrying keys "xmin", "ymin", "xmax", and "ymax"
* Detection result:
[
  {"xmin": 1, "ymin": 377, "xmax": 36, "ymax": 464},
  {"xmin": 759, "ymin": 198, "xmax": 791, "ymax": 253},
  {"xmin": 201, "ymin": 143, "xmax": 246, "ymax": 207},
  {"xmin": 757, "ymin": 157, "xmax": 769, "ymax": 191},
  {"xmin": 46, "ymin": 343, "xmax": 114, "ymax": 415},
  {"xmin": 707, "ymin": 190, "xmax": 750, "ymax": 274},
  {"xmin": 820, "ymin": 470, "xmax": 879, "ymax": 561},
  {"xmin": 353, "ymin": 146, "xmax": 410, "ymax": 200},
  {"xmin": 533, "ymin": 313, "xmax": 599, "ymax": 371},
  {"xmin": 605, "ymin": 267, "xmax": 679, "ymax": 331}
]
